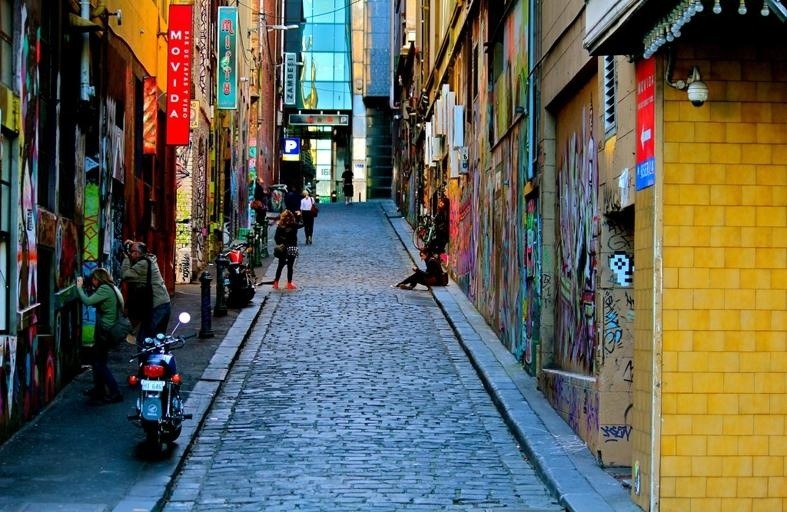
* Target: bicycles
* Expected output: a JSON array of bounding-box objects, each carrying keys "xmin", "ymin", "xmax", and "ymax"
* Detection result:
[
  {"xmin": 413, "ymin": 212, "xmax": 435, "ymax": 251},
  {"xmin": 249, "ymin": 221, "xmax": 268, "ymax": 267}
]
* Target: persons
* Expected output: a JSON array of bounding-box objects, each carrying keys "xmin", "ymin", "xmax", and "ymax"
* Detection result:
[
  {"xmin": 434, "ymin": 197, "xmax": 450, "ymax": 250},
  {"xmin": 76, "ymin": 267, "xmax": 124, "ymax": 405},
  {"xmin": 284, "ymin": 187, "xmax": 302, "ymax": 230},
  {"xmin": 273, "ymin": 210, "xmax": 304, "ymax": 291},
  {"xmin": 254, "ymin": 177, "xmax": 267, "ymax": 230},
  {"xmin": 120, "ymin": 238, "xmax": 172, "ymax": 390},
  {"xmin": 389, "ymin": 248, "xmax": 443, "ymax": 290},
  {"xmin": 342, "ymin": 164, "xmax": 353, "ymax": 206},
  {"xmin": 300, "ymin": 190, "xmax": 315, "ymax": 245}
]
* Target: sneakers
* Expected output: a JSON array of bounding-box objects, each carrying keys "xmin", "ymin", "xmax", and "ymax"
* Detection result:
[
  {"xmin": 286, "ymin": 282, "xmax": 296, "ymax": 290},
  {"xmin": 273, "ymin": 280, "xmax": 279, "ymax": 289},
  {"xmin": 83, "ymin": 386, "xmax": 123, "ymax": 403}
]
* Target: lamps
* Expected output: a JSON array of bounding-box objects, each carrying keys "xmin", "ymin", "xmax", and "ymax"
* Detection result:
[{"xmin": 665, "ymin": 48, "xmax": 709, "ymax": 108}]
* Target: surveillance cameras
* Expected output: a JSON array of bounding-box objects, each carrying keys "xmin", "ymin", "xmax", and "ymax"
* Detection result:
[{"xmin": 687, "ymin": 81, "xmax": 711, "ymax": 109}]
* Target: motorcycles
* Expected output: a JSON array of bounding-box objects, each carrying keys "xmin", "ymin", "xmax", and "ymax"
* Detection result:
[
  {"xmin": 125, "ymin": 312, "xmax": 196, "ymax": 452},
  {"xmin": 218, "ymin": 230, "xmax": 257, "ymax": 309}
]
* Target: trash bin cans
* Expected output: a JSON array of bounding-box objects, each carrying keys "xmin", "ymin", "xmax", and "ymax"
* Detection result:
[{"xmin": 268, "ymin": 184, "xmax": 287, "ymax": 212}]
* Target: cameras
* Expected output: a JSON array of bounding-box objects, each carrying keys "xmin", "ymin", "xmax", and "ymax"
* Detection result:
[{"xmin": 123, "ymin": 242, "xmax": 130, "ymax": 254}]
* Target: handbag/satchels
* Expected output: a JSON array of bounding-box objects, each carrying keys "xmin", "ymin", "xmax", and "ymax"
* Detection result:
[
  {"xmin": 100, "ymin": 311, "xmax": 134, "ymax": 351},
  {"xmin": 126, "ymin": 256, "xmax": 155, "ymax": 322},
  {"xmin": 310, "ymin": 196, "xmax": 318, "ymax": 217}
]
